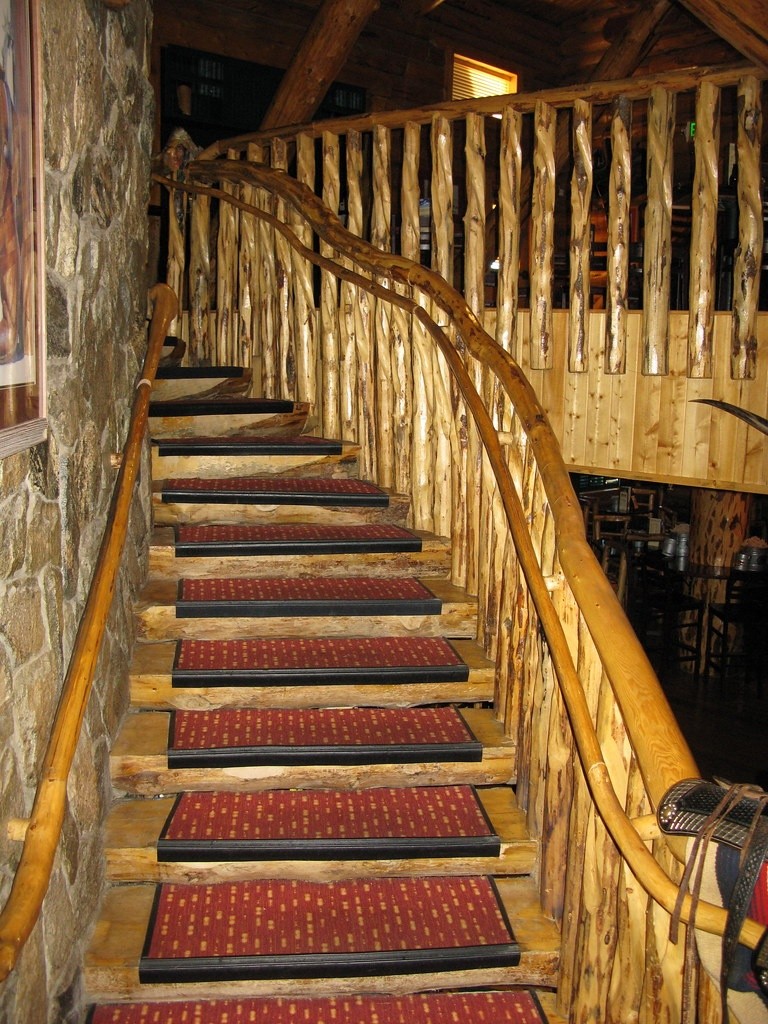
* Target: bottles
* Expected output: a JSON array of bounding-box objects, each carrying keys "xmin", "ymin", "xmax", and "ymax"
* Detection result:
[
  {"xmin": 598, "ymin": 536, "xmax": 618, "ymax": 571},
  {"xmin": 579, "ymin": 475, "xmax": 604, "ymax": 488},
  {"xmin": 611, "ymin": 493, "xmax": 618, "ymax": 512}
]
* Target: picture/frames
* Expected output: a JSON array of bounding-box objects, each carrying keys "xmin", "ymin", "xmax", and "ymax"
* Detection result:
[{"xmin": 0, "ymin": 0, "xmax": 49, "ymax": 458}]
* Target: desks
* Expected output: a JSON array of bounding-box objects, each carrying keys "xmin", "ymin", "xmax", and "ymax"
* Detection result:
[{"xmin": 674, "ymin": 180, "xmax": 768, "ymax": 310}]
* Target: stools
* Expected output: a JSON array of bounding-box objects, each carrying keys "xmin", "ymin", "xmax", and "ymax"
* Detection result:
[
  {"xmin": 709, "ymin": 571, "xmax": 766, "ymax": 697},
  {"xmin": 630, "ymin": 554, "xmax": 703, "ymax": 682}
]
